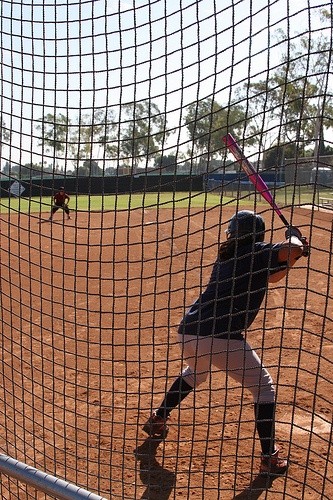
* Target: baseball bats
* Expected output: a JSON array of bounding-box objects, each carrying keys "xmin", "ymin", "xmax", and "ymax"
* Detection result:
[{"xmin": 221, "ymin": 132, "xmax": 309, "ymax": 257}]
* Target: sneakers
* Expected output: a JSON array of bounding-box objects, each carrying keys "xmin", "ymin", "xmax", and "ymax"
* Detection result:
[
  {"xmin": 143, "ymin": 411, "xmax": 169, "ymax": 435},
  {"xmin": 259, "ymin": 449, "xmax": 289, "ymax": 474}
]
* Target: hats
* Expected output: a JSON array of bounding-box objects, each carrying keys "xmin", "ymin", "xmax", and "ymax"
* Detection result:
[{"xmin": 59, "ymin": 187, "xmax": 65, "ymax": 190}]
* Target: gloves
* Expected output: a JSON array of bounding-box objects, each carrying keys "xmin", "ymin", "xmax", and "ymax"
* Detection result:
[{"xmin": 295, "ymin": 237, "xmax": 311, "ymax": 260}]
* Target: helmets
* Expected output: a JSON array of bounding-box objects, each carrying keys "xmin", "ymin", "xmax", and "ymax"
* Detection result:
[{"xmin": 228, "ymin": 208, "xmax": 266, "ymax": 243}]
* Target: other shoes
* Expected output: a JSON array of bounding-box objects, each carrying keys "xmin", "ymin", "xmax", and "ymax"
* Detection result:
[
  {"xmin": 67, "ymin": 216, "xmax": 71, "ymax": 219},
  {"xmin": 49, "ymin": 217, "xmax": 51, "ymax": 220}
]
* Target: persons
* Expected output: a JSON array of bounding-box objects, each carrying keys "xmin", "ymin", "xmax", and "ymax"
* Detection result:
[
  {"xmin": 141, "ymin": 209, "xmax": 305, "ymax": 476},
  {"xmin": 48, "ymin": 187, "xmax": 71, "ymax": 221}
]
